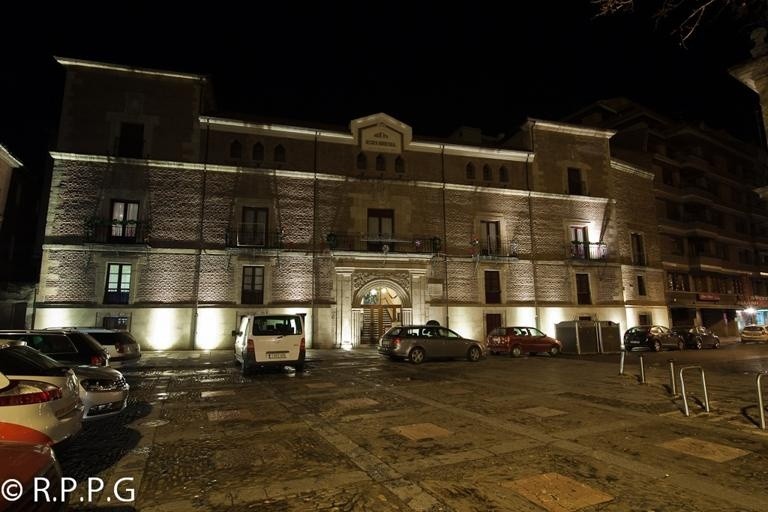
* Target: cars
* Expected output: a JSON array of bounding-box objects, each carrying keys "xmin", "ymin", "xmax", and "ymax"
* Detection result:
[
  {"xmin": 623, "ymin": 324, "xmax": 687, "ymax": 353},
  {"xmin": 0, "ymin": 324, "xmax": 144, "ymax": 511},
  {"xmin": 484, "ymin": 326, "xmax": 564, "ymax": 358},
  {"xmin": 738, "ymin": 325, "xmax": 768, "ymax": 345},
  {"xmin": 669, "ymin": 324, "xmax": 721, "ymax": 351}
]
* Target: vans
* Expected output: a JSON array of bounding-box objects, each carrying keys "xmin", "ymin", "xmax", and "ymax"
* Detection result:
[
  {"xmin": 230, "ymin": 312, "xmax": 307, "ymax": 375},
  {"xmin": 377, "ymin": 325, "xmax": 489, "ymax": 364}
]
